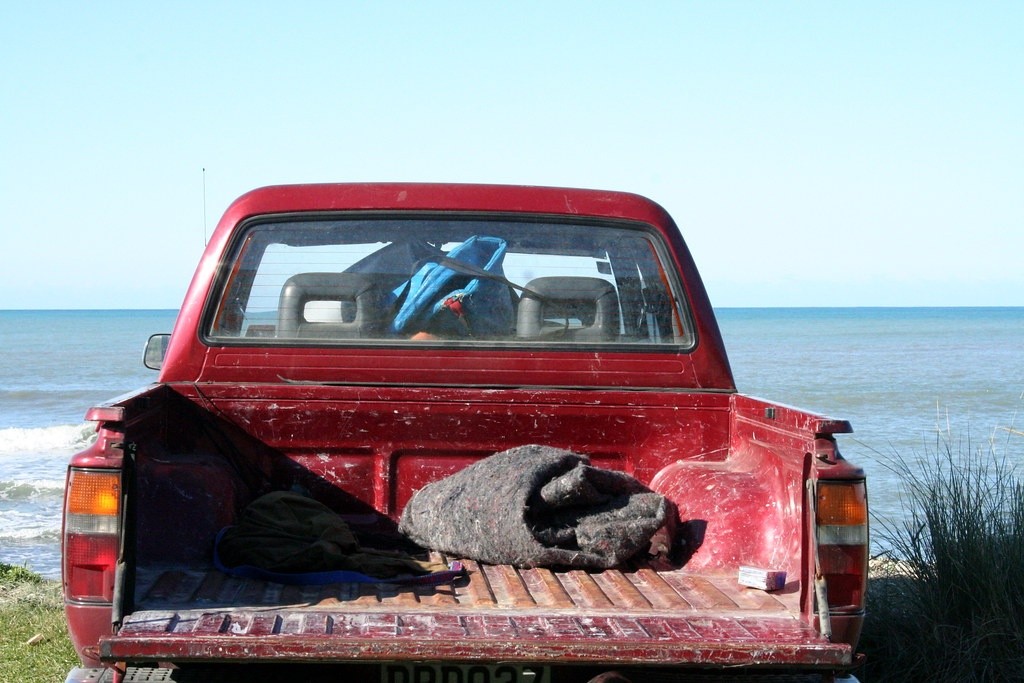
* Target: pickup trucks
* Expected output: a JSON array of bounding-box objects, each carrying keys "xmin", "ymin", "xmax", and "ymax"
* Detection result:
[{"xmin": 58, "ymin": 181, "xmax": 873, "ymax": 683}]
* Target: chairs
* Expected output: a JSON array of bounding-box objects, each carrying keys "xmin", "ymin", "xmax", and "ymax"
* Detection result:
[
  {"xmin": 275, "ymin": 271, "xmax": 387, "ymax": 340},
  {"xmin": 517, "ymin": 277, "xmax": 622, "ymax": 343}
]
